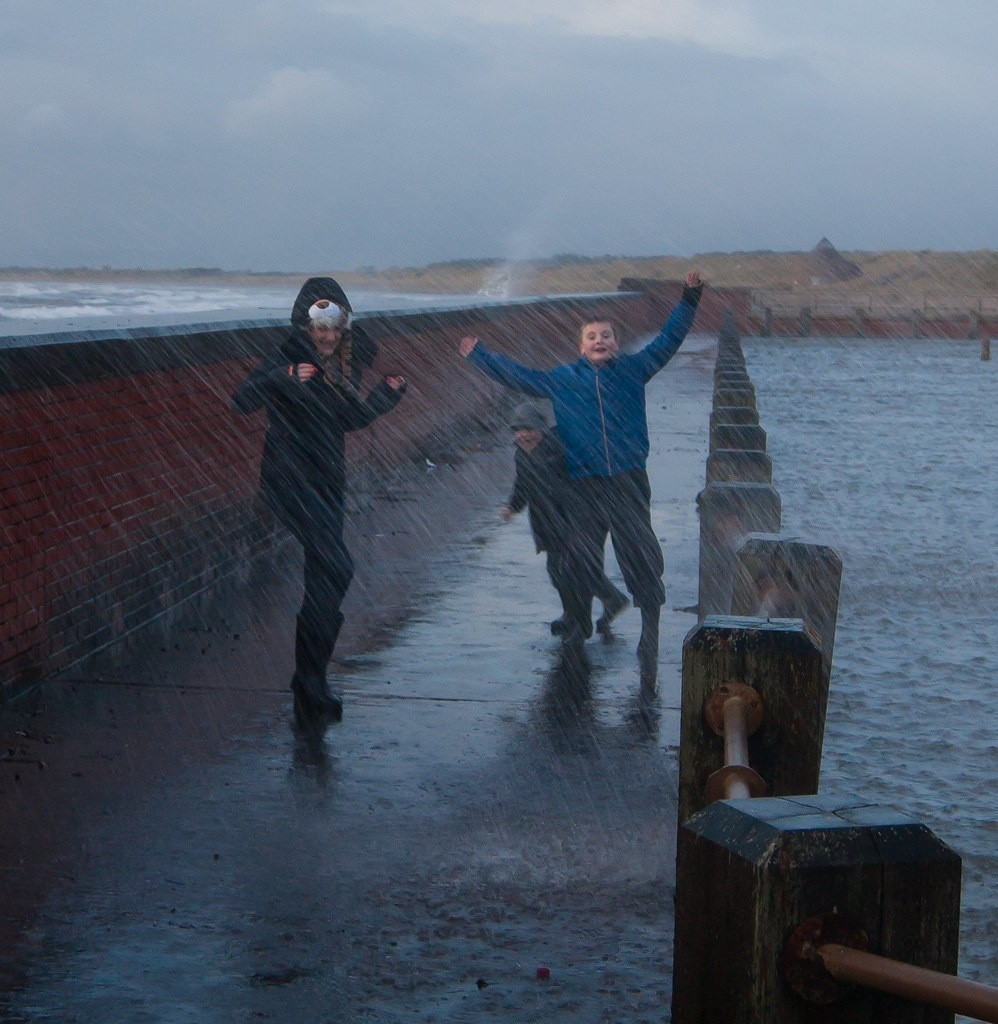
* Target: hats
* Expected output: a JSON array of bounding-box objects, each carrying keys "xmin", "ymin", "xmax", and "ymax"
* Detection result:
[{"xmin": 510, "ymin": 401, "xmax": 550, "ymax": 432}]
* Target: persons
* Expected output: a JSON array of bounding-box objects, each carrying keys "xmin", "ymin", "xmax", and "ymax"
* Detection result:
[
  {"xmin": 231, "ymin": 275, "xmax": 409, "ymax": 719},
  {"xmin": 458, "ymin": 269, "xmax": 706, "ymax": 656},
  {"xmin": 497, "ymin": 401, "xmax": 630, "ymax": 634}
]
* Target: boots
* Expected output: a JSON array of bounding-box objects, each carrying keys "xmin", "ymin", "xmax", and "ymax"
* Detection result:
[
  {"xmin": 637, "ymin": 605, "xmax": 660, "ymax": 656},
  {"xmin": 551, "ymin": 587, "xmax": 593, "ymax": 644},
  {"xmin": 596, "ymin": 582, "xmax": 630, "ymax": 626},
  {"xmin": 290, "ymin": 611, "xmax": 343, "ymax": 709}
]
